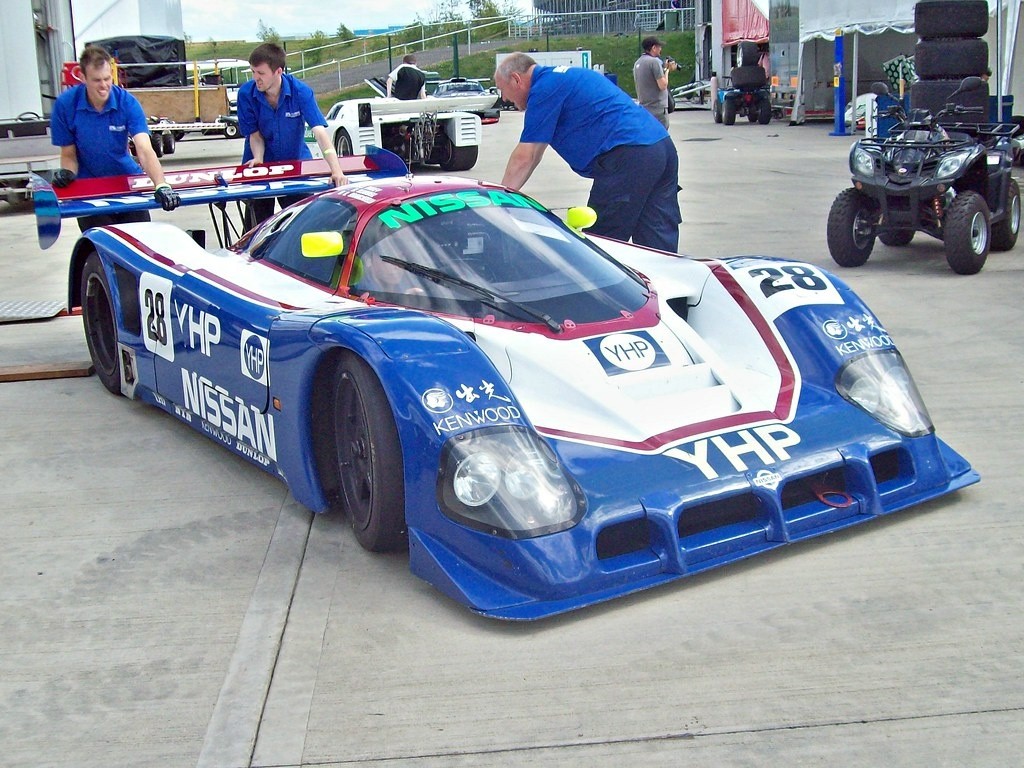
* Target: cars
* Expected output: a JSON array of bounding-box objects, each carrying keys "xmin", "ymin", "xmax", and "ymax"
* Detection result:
[
  {"xmin": 314, "ymin": 95, "xmax": 500, "ymax": 170},
  {"xmin": 67, "ymin": 171, "xmax": 981, "ymax": 623},
  {"xmin": 432, "ymin": 77, "xmax": 486, "ymax": 98}
]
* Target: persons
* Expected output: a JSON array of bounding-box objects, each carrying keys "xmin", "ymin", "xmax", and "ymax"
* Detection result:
[
  {"xmin": 632, "ymin": 36, "xmax": 677, "ymax": 130},
  {"xmin": 236, "ymin": 43, "xmax": 349, "ymax": 236},
  {"xmin": 386, "ymin": 55, "xmax": 425, "ymax": 100},
  {"xmin": 50, "ymin": 44, "xmax": 181, "ymax": 234},
  {"xmin": 493, "ymin": 51, "xmax": 683, "ymax": 253},
  {"xmin": 981, "ymin": 69, "xmax": 992, "ymax": 80},
  {"xmin": 654, "ymin": 57, "xmax": 664, "ymax": 70}
]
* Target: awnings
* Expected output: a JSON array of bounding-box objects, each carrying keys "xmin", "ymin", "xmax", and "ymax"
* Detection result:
[{"xmin": 185, "ymin": 59, "xmax": 250, "ymax": 70}]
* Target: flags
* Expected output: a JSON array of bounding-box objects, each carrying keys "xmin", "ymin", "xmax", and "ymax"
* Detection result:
[
  {"xmin": 882, "ymin": 56, "xmax": 913, "ymax": 93},
  {"xmin": 903, "ymin": 56, "xmax": 918, "ymax": 85}
]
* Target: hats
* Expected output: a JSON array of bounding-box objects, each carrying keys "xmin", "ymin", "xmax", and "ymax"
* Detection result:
[{"xmin": 642, "ymin": 36, "xmax": 667, "ymax": 48}]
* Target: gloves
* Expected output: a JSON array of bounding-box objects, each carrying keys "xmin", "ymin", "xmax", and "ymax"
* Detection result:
[
  {"xmin": 52, "ymin": 169, "xmax": 75, "ymax": 188},
  {"xmin": 154, "ymin": 182, "xmax": 181, "ymax": 211}
]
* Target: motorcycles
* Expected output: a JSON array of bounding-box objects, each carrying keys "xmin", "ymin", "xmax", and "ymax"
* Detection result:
[
  {"xmin": 825, "ymin": 76, "xmax": 1021, "ymax": 275},
  {"xmin": 712, "ymin": 76, "xmax": 772, "ymax": 127}
]
